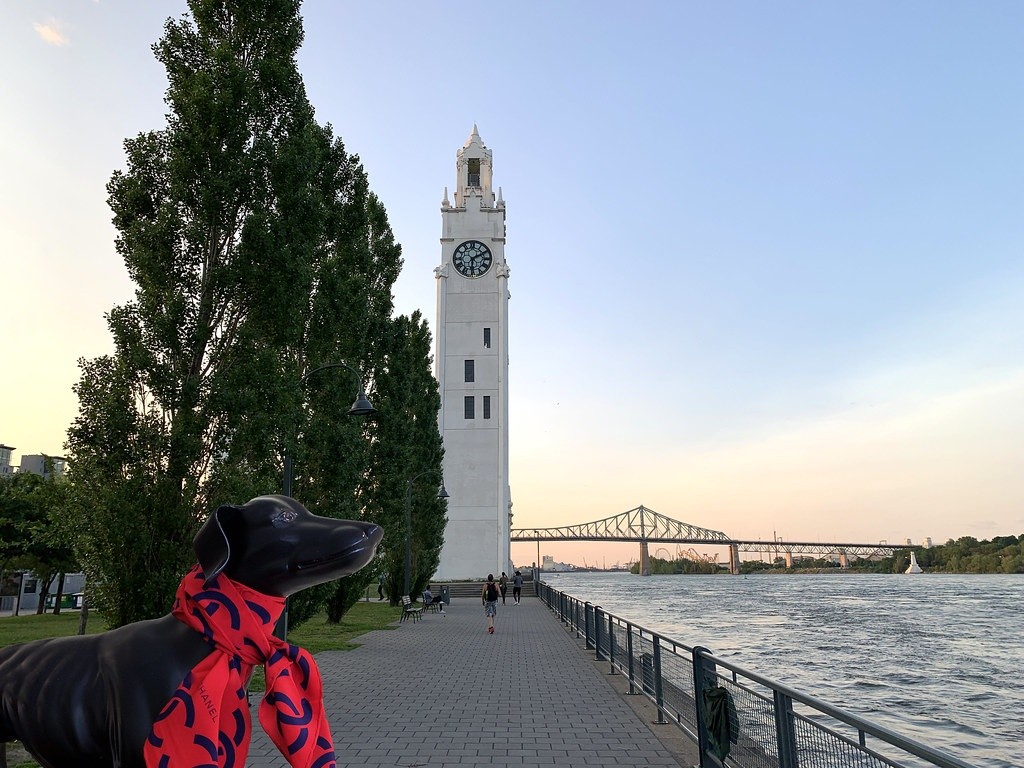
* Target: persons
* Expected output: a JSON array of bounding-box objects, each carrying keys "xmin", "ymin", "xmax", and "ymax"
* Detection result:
[
  {"xmin": 482, "ymin": 574, "xmax": 502, "ymax": 634},
  {"xmin": 377, "ymin": 572, "xmax": 389, "ymax": 601},
  {"xmin": 499, "ymin": 572, "xmax": 508, "ymax": 606},
  {"xmin": 425, "ymin": 585, "xmax": 446, "ymax": 614},
  {"xmin": 513, "ymin": 570, "xmax": 523, "ymax": 606}
]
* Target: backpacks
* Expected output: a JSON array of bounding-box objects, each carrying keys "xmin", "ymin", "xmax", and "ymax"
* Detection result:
[{"xmin": 486, "ymin": 582, "xmax": 498, "ymax": 602}]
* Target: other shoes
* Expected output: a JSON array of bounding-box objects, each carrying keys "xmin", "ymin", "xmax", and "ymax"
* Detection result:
[
  {"xmin": 514, "ymin": 602, "xmax": 520, "ymax": 605},
  {"xmin": 439, "ymin": 601, "xmax": 445, "ymax": 604},
  {"xmin": 489, "ymin": 626, "xmax": 494, "ymax": 634},
  {"xmin": 440, "ymin": 610, "xmax": 446, "ymax": 614}
]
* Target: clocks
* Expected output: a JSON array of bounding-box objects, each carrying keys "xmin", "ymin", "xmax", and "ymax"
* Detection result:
[{"xmin": 453, "ymin": 240, "xmax": 492, "ymax": 278}]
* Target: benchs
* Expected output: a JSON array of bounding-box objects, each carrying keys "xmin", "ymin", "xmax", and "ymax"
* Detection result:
[
  {"xmin": 422, "ymin": 592, "xmax": 439, "ymax": 614},
  {"xmin": 399, "ymin": 595, "xmax": 422, "ymax": 623}
]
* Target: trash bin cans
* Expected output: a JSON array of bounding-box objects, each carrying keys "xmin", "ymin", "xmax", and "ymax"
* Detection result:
[{"xmin": 438, "ymin": 585, "xmax": 450, "ymax": 605}]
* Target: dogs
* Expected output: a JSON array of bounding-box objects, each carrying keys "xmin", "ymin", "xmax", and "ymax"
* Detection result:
[{"xmin": 0, "ymin": 493, "xmax": 389, "ymax": 768}]
